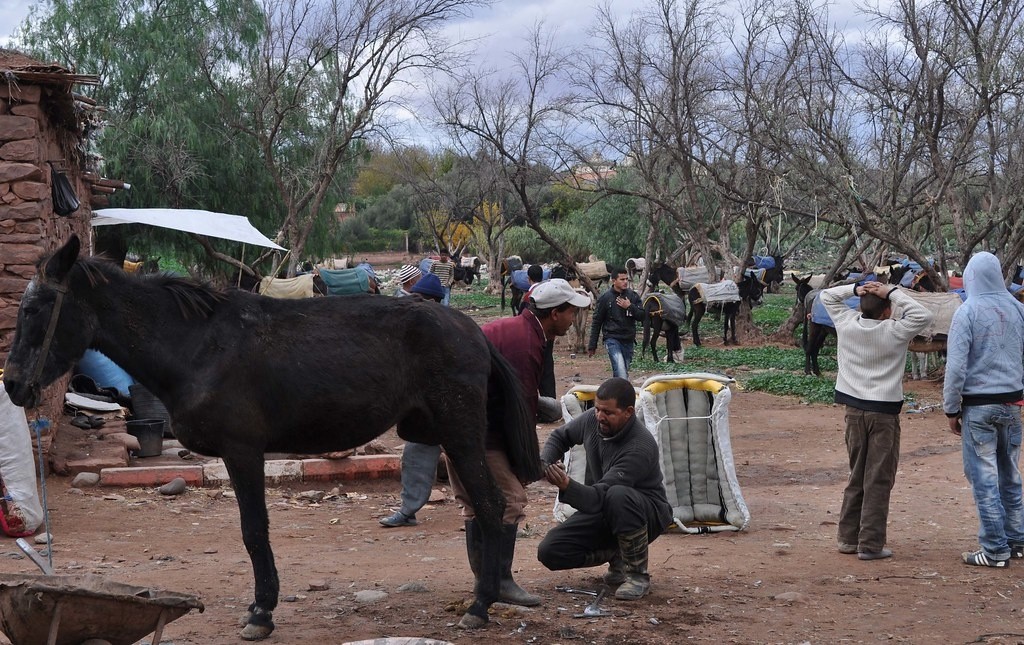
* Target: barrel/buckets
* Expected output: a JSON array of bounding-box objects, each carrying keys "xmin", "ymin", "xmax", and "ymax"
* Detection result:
[{"xmin": 126, "ymin": 418, "xmax": 165, "ymax": 456}]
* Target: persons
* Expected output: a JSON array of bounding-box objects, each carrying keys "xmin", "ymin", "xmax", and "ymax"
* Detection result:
[
  {"xmin": 517, "ymin": 377, "xmax": 674, "ymax": 600},
  {"xmin": 427, "ymin": 248, "xmax": 455, "ymax": 308},
  {"xmin": 819, "ymin": 282, "xmax": 933, "ymax": 560},
  {"xmin": 379, "ymin": 273, "xmax": 446, "ymax": 523},
  {"xmin": 943, "ymin": 251, "xmax": 1024, "ymax": 567},
  {"xmin": 918, "ymin": 275, "xmax": 936, "ymax": 293},
  {"xmin": 588, "ymin": 268, "xmax": 646, "ymax": 381},
  {"xmin": 440, "ymin": 278, "xmax": 590, "ymax": 606},
  {"xmin": 518, "ymin": 266, "xmax": 556, "ymax": 400},
  {"xmin": 394, "ymin": 265, "xmax": 422, "ymax": 297}
]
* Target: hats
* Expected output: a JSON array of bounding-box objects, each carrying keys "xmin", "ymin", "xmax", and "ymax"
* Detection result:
[
  {"xmin": 528, "ymin": 278, "xmax": 591, "ymax": 308},
  {"xmin": 411, "ymin": 273, "xmax": 445, "ymax": 298},
  {"xmin": 400, "ymin": 265, "xmax": 420, "ymax": 283}
]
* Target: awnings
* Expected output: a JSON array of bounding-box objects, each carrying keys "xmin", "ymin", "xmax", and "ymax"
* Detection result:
[{"xmin": 93, "ymin": 208, "xmax": 291, "ymax": 295}]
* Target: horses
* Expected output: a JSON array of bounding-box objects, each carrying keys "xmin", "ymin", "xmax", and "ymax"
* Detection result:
[
  {"xmin": 686, "ymin": 271, "xmax": 764, "ymax": 348},
  {"xmin": 3, "ymin": 231, "xmax": 545, "ymax": 641},
  {"xmin": 501, "ymin": 255, "xmax": 646, "ymax": 354},
  {"xmin": 646, "ymin": 262, "xmax": 725, "ymax": 307},
  {"xmin": 451, "ymin": 256, "xmax": 481, "ymax": 286},
  {"xmin": 641, "ymin": 293, "xmax": 689, "ymax": 364},
  {"xmin": 791, "ymin": 259, "xmax": 948, "ymax": 380},
  {"xmin": 748, "ymin": 255, "xmax": 784, "ymax": 289}
]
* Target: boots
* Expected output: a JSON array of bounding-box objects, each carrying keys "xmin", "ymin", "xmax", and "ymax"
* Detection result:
[
  {"xmin": 615, "ymin": 522, "xmax": 651, "ymax": 600},
  {"xmin": 464, "ymin": 520, "xmax": 481, "ymax": 574},
  {"xmin": 494, "ymin": 523, "xmax": 542, "ymax": 605},
  {"xmin": 584, "ymin": 545, "xmax": 624, "ymax": 584}
]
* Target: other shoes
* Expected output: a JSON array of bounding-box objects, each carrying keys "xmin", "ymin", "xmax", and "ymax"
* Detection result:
[{"xmin": 858, "ymin": 550, "xmax": 891, "ymax": 560}]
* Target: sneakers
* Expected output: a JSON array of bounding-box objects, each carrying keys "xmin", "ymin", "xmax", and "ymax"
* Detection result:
[
  {"xmin": 1010, "ymin": 547, "xmax": 1023, "ymax": 558},
  {"xmin": 962, "ymin": 550, "xmax": 1009, "ymax": 567},
  {"xmin": 379, "ymin": 511, "xmax": 417, "ymax": 526}
]
